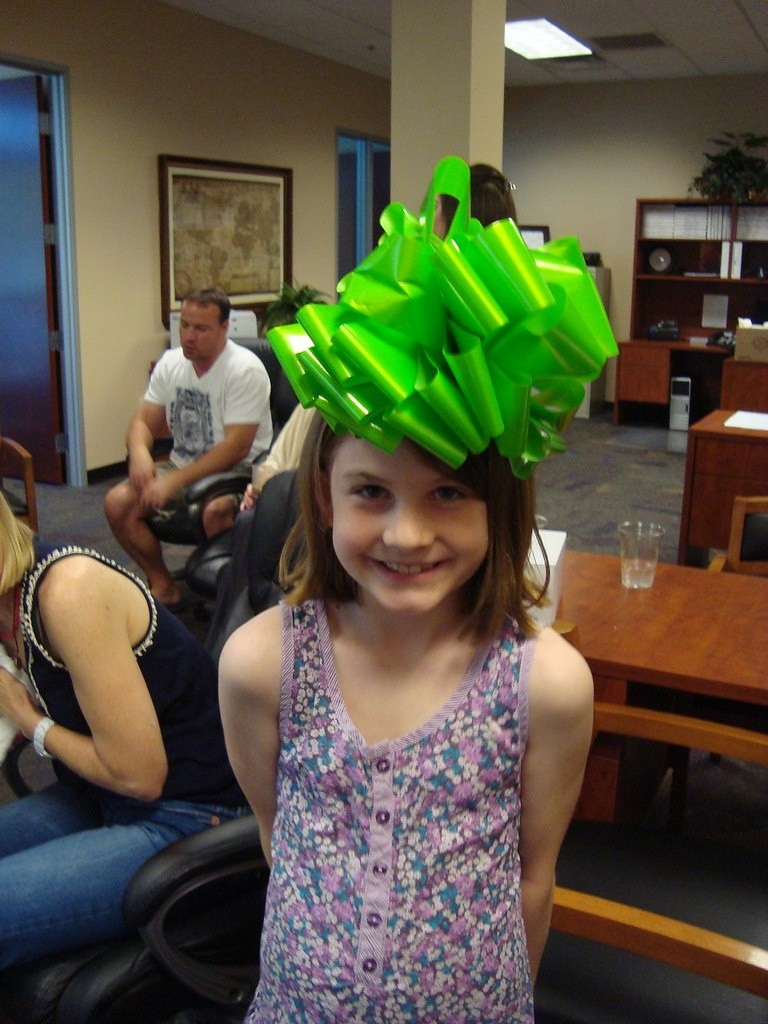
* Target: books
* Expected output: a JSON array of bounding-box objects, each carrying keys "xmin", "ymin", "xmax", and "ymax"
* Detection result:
[{"xmin": 641, "ymin": 204, "xmax": 768, "ymax": 279}]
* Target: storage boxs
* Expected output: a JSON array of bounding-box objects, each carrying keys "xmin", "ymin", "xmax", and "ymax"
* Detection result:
[{"xmin": 735, "ymin": 324, "xmax": 768, "ymax": 364}]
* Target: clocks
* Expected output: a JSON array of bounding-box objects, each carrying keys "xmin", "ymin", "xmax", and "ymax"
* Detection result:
[{"xmin": 647, "ymin": 241, "xmax": 680, "ymax": 275}]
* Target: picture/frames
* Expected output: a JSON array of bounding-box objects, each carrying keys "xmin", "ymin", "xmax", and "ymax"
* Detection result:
[
  {"xmin": 157, "ymin": 155, "xmax": 292, "ymax": 329},
  {"xmin": 519, "ymin": 225, "xmax": 549, "ymax": 248}
]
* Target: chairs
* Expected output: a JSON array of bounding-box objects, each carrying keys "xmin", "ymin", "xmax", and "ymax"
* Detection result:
[
  {"xmin": 0, "ymin": 468, "xmax": 304, "ymax": 1024},
  {"xmin": 142, "ymin": 337, "xmax": 298, "ymax": 596},
  {"xmin": 0, "ymin": 435, "xmax": 39, "ymax": 667},
  {"xmin": 664, "ymin": 495, "xmax": 768, "ymax": 850}
]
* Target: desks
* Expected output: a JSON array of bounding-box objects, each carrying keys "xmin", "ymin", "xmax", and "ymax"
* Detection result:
[
  {"xmin": 551, "ymin": 550, "xmax": 768, "ymax": 827},
  {"xmin": 719, "ymin": 357, "xmax": 768, "ymax": 413},
  {"xmin": 677, "ymin": 411, "xmax": 768, "ymax": 568}
]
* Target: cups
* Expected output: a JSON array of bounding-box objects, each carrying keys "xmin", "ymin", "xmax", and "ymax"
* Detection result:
[{"xmin": 618, "ymin": 519, "xmax": 666, "ymax": 590}]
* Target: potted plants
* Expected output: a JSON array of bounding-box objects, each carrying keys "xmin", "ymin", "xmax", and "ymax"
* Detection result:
[{"xmin": 692, "ymin": 130, "xmax": 768, "ymax": 204}]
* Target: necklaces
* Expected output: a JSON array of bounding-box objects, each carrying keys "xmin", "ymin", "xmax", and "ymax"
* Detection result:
[{"xmin": 0, "ymin": 584, "xmax": 21, "ymax": 671}]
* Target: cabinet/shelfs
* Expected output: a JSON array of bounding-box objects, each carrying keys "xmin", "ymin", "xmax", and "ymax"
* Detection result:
[
  {"xmin": 613, "ymin": 197, "xmax": 768, "ymax": 427},
  {"xmin": 530, "ymin": 267, "xmax": 612, "ymax": 420}
]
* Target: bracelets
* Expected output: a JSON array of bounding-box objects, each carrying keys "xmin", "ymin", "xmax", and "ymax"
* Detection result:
[{"xmin": 34, "ymin": 718, "xmax": 56, "ymax": 760}]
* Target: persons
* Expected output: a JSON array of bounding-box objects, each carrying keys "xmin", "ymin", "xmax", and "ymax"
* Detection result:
[
  {"xmin": 435, "ymin": 164, "xmax": 517, "ymax": 242},
  {"xmin": 0, "ymin": 492, "xmax": 254, "ymax": 970},
  {"xmin": 101, "ymin": 289, "xmax": 315, "ymax": 622},
  {"xmin": 215, "ymin": 158, "xmax": 618, "ymax": 1024}
]
